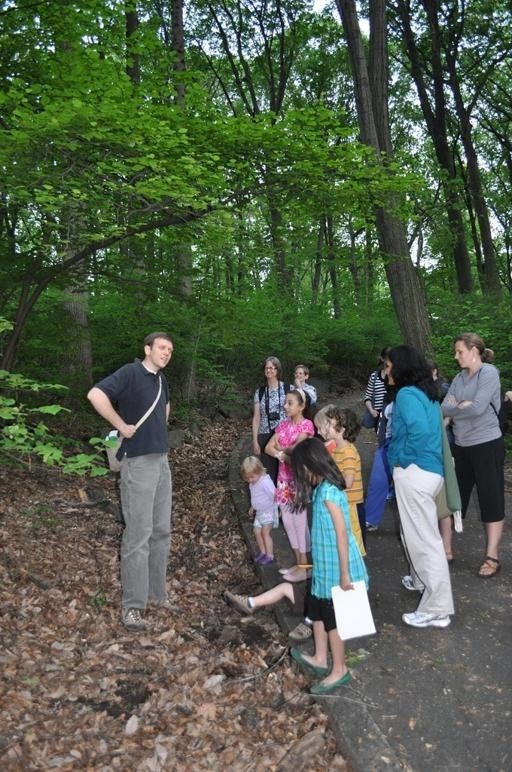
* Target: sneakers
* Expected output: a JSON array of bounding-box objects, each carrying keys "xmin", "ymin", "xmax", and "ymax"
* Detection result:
[
  {"xmin": 401, "ymin": 610, "xmax": 452, "ymax": 630},
  {"xmin": 287, "ymin": 619, "xmax": 313, "ymax": 643},
  {"xmin": 363, "ymin": 520, "xmax": 380, "ymax": 534},
  {"xmin": 119, "ymin": 606, "xmax": 148, "ymax": 633},
  {"xmin": 222, "ymin": 589, "xmax": 257, "ymax": 617},
  {"xmin": 147, "ymin": 600, "xmax": 180, "ymax": 618},
  {"xmin": 402, "ymin": 574, "xmax": 425, "ymax": 592}
]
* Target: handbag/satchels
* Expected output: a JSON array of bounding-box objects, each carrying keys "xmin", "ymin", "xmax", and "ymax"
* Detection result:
[
  {"xmin": 361, "ymin": 406, "xmax": 376, "ymax": 430},
  {"xmin": 104, "ymin": 428, "xmax": 127, "ymax": 473}
]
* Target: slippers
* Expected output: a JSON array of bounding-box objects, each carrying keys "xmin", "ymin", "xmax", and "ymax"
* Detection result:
[
  {"xmin": 444, "ymin": 550, "xmax": 454, "ymax": 565},
  {"xmin": 478, "ymin": 555, "xmax": 504, "ymax": 578}
]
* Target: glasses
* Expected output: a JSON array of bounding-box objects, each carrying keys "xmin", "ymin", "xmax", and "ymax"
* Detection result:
[{"xmin": 263, "ymin": 366, "xmax": 277, "ymax": 370}]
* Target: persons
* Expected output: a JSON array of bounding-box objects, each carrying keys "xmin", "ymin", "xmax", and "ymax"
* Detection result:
[
  {"xmin": 264, "ymin": 388, "xmax": 315, "ymax": 582},
  {"xmin": 287, "ymin": 365, "xmax": 318, "ymax": 406},
  {"xmin": 439, "ymin": 331, "xmax": 505, "ymax": 577},
  {"xmin": 252, "ymin": 354, "xmax": 292, "ymax": 480},
  {"xmin": 289, "ymin": 438, "xmax": 370, "ymax": 694},
  {"xmin": 355, "ymin": 340, "xmax": 463, "ymax": 564},
  {"xmin": 326, "ymin": 408, "xmax": 370, "ymax": 560},
  {"xmin": 313, "ymin": 402, "xmax": 335, "ymax": 448},
  {"xmin": 382, "ymin": 346, "xmax": 457, "ymax": 633},
  {"xmin": 221, "ymin": 577, "xmax": 313, "ymax": 641},
  {"xmin": 86, "ymin": 328, "xmax": 183, "ymax": 628},
  {"xmin": 239, "ymin": 456, "xmax": 277, "ymax": 567}
]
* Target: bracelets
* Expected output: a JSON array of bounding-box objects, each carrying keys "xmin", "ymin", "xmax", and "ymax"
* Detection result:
[{"xmin": 278, "ymin": 450, "xmax": 284, "ymax": 464}]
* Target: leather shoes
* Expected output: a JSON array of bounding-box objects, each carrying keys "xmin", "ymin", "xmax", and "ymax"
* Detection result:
[
  {"xmin": 253, "ymin": 552, "xmax": 266, "ymax": 563},
  {"xmin": 278, "ymin": 563, "xmax": 300, "ymax": 576},
  {"xmin": 258, "ymin": 554, "xmax": 275, "ymax": 566},
  {"xmin": 283, "ymin": 567, "xmax": 313, "ymax": 584},
  {"xmin": 309, "ymin": 669, "xmax": 353, "ymax": 695},
  {"xmin": 290, "ymin": 647, "xmax": 329, "ymax": 678}
]
